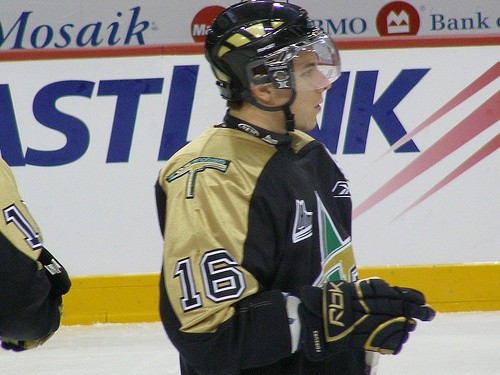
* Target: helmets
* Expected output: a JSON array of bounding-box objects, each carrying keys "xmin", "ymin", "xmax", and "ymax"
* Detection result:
[{"xmin": 205, "ymin": 0, "xmax": 309, "ymax": 102}]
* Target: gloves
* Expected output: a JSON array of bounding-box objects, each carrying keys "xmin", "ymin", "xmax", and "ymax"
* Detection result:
[{"xmin": 298, "ymin": 279, "xmax": 435, "ymax": 361}]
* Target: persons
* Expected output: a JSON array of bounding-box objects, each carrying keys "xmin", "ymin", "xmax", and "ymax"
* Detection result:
[
  {"xmin": 0, "ymin": 155, "xmax": 71, "ymax": 353},
  {"xmin": 155, "ymin": 0, "xmax": 435, "ymax": 375}
]
